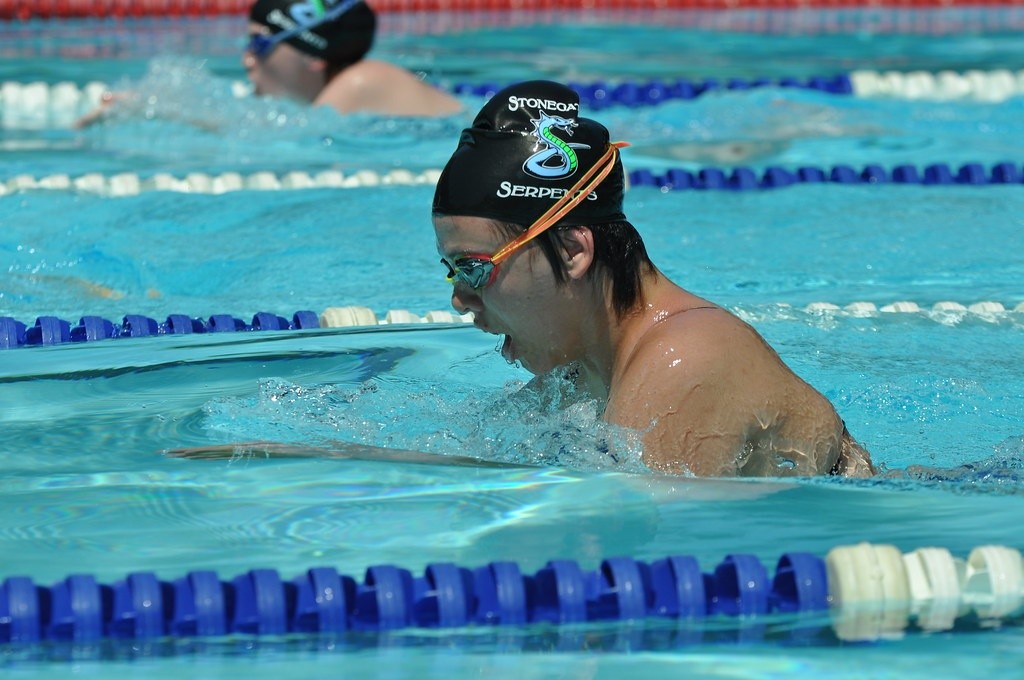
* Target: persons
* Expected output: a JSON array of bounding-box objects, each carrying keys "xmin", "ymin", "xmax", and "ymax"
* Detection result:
[
  {"xmin": 243, "ymin": 0, "xmax": 463, "ymax": 118},
  {"xmin": 432, "ymin": 79, "xmax": 876, "ymax": 479}
]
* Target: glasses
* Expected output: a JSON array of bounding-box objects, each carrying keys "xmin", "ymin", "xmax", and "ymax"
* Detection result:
[
  {"xmin": 248, "ymin": 0, "xmax": 363, "ymax": 63},
  {"xmin": 440, "ymin": 139, "xmax": 631, "ymax": 288}
]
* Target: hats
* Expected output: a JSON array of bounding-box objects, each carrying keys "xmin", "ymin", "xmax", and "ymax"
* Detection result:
[
  {"xmin": 431, "ymin": 80, "xmax": 628, "ymax": 223},
  {"xmin": 248, "ymin": 0, "xmax": 377, "ymax": 69}
]
